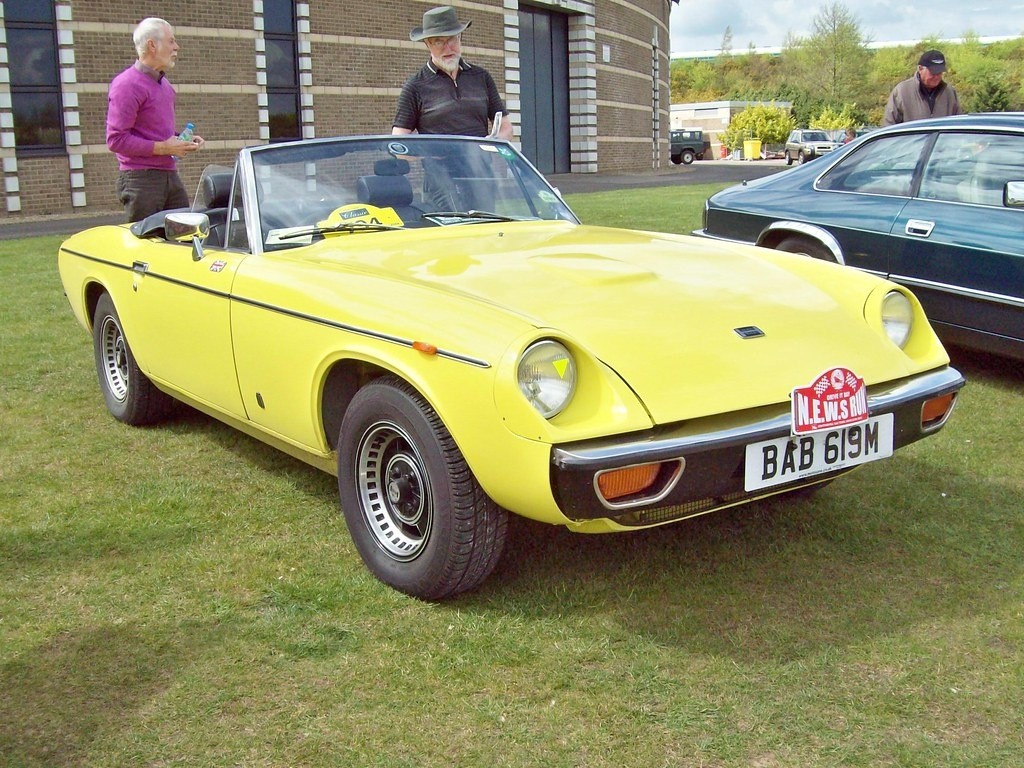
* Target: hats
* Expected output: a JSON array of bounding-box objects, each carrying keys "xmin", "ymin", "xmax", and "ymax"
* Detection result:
[
  {"xmin": 409, "ymin": 6, "xmax": 472, "ymax": 41},
  {"xmin": 918, "ymin": 50, "xmax": 947, "ymax": 75}
]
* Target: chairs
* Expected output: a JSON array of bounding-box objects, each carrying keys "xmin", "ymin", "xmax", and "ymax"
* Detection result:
[
  {"xmin": 199, "ymin": 173, "xmax": 277, "ymax": 251},
  {"xmin": 357, "ymin": 158, "xmax": 440, "ymax": 228}
]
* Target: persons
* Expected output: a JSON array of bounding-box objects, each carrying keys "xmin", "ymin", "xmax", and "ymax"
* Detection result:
[
  {"xmin": 106, "ymin": 17, "xmax": 206, "ymax": 222},
  {"xmin": 884, "ymin": 50, "xmax": 963, "ymax": 127},
  {"xmin": 844, "ymin": 128, "xmax": 856, "ymax": 144},
  {"xmin": 391, "ymin": 6, "xmax": 513, "ymax": 212}
]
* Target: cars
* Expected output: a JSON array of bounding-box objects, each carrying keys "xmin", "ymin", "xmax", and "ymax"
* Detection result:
[
  {"xmin": 690, "ymin": 110, "xmax": 1024, "ymax": 364},
  {"xmin": 670, "ymin": 131, "xmax": 705, "ymax": 164}
]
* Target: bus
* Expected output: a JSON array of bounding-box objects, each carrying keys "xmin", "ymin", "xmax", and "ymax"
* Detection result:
[{"xmin": 838, "ymin": 128, "xmax": 872, "ymax": 145}]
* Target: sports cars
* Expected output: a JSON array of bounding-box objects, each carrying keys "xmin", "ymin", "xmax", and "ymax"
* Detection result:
[{"xmin": 59, "ymin": 135, "xmax": 969, "ymax": 605}]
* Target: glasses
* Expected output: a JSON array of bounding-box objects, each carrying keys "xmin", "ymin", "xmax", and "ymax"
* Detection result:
[{"xmin": 428, "ymin": 35, "xmax": 460, "ymax": 48}]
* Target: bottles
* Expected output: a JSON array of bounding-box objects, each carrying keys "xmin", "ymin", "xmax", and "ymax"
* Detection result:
[{"xmin": 170, "ymin": 123, "xmax": 196, "ymax": 160}]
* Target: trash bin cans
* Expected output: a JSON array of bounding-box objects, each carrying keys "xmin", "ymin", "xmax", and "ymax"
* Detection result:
[{"xmin": 743, "ymin": 141, "xmax": 762, "ymax": 159}]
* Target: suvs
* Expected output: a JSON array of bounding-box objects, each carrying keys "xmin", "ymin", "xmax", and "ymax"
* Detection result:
[{"xmin": 785, "ymin": 129, "xmax": 839, "ymax": 166}]
